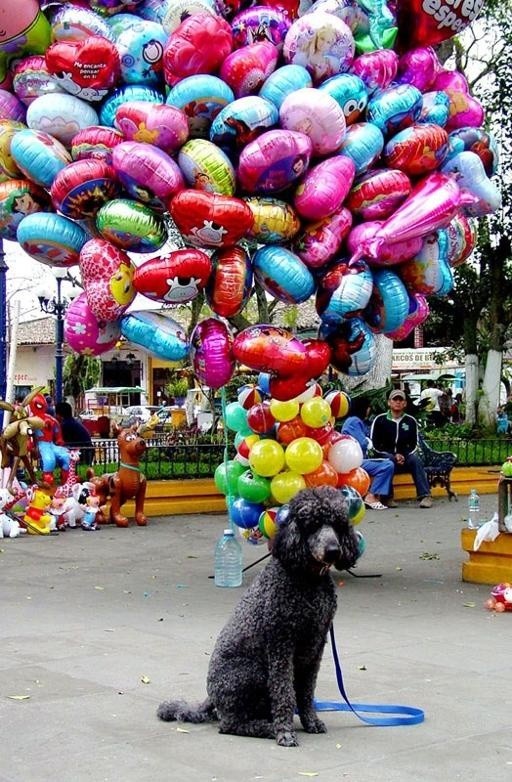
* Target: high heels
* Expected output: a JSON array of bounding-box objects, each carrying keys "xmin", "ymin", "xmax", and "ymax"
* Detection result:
[{"xmin": 364, "ymin": 497, "xmax": 388, "ymax": 510}]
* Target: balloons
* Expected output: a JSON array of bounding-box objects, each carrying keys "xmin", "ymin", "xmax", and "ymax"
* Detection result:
[
  {"xmin": 0, "ymin": 1, "xmax": 506, "ymax": 417},
  {"xmin": 213, "ymin": 397, "xmax": 371, "ymax": 560}
]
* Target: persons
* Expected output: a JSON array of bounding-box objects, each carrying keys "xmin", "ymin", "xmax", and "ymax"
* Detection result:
[
  {"xmin": 342, "ymin": 380, "xmax": 462, "ymax": 510},
  {"xmin": 41, "ymin": 393, "xmax": 95, "ymax": 465}
]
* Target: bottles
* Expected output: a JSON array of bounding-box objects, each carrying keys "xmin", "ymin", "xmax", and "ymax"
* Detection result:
[{"xmin": 467, "ymin": 488, "xmax": 481, "ymax": 529}]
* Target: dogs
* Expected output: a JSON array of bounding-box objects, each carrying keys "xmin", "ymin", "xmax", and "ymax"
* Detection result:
[{"xmin": 156, "ymin": 485, "xmax": 361, "ymax": 747}]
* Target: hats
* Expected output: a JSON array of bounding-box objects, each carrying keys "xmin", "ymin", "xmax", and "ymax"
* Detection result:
[{"xmin": 389, "ymin": 389, "xmax": 406, "ymax": 400}]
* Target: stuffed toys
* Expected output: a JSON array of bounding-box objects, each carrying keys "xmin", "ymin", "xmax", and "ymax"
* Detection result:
[{"xmin": 484, "ymin": 583, "xmax": 512, "ymax": 613}]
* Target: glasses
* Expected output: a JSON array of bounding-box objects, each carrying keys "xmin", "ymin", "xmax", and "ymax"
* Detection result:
[{"xmin": 393, "ymin": 397, "xmax": 403, "ymax": 401}]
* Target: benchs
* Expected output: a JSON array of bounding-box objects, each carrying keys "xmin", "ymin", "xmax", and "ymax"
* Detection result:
[
  {"xmin": 461, "ymin": 528, "xmax": 512, "ymax": 588},
  {"xmin": 101, "ymin": 466, "xmax": 502, "ymax": 520}
]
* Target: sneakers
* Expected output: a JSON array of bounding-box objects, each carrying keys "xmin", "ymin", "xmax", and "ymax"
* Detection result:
[
  {"xmin": 383, "ymin": 498, "xmax": 398, "ymax": 507},
  {"xmin": 419, "ymin": 496, "xmax": 433, "ymax": 508}
]
